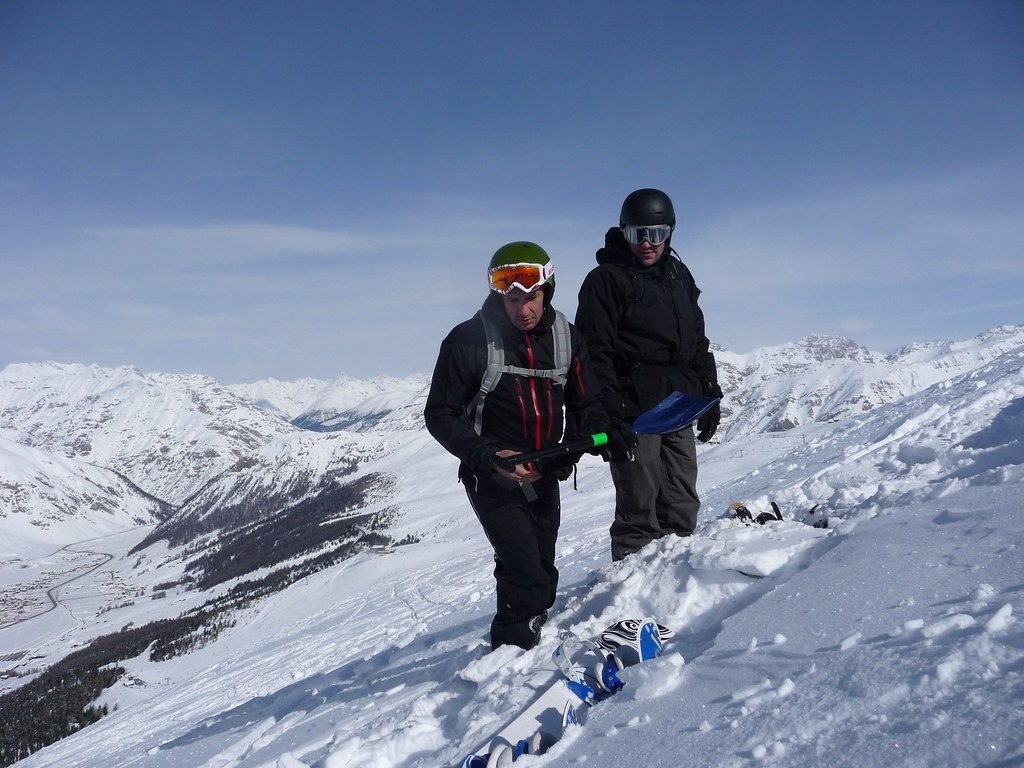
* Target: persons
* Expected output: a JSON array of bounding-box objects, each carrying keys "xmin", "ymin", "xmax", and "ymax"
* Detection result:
[
  {"xmin": 574, "ymin": 188, "xmax": 724, "ymax": 563},
  {"xmin": 424, "ymin": 242, "xmax": 598, "ymax": 651}
]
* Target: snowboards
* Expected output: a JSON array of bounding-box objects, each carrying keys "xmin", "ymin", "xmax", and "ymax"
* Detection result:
[{"xmin": 460, "ymin": 618, "xmax": 675, "ymax": 768}]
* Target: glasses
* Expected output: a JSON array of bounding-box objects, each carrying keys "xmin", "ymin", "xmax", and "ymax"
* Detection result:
[
  {"xmin": 620, "ymin": 225, "xmax": 671, "ymax": 246},
  {"xmin": 489, "ymin": 263, "xmax": 546, "ymax": 295}
]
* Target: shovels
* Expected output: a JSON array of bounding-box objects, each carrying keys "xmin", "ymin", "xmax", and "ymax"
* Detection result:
[{"xmin": 490, "ymin": 390, "xmax": 720, "ymax": 473}]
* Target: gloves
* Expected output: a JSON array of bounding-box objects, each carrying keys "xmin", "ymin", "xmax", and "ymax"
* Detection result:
[
  {"xmin": 578, "ymin": 401, "xmax": 639, "ymax": 463},
  {"xmin": 695, "ymin": 395, "xmax": 721, "ymax": 443},
  {"xmin": 539, "ymin": 446, "xmax": 572, "ymax": 480}
]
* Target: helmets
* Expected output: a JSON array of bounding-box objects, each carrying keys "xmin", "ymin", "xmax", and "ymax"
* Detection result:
[
  {"xmin": 487, "ymin": 241, "xmax": 554, "ymax": 302},
  {"xmin": 620, "ymin": 189, "xmax": 676, "ymax": 245}
]
